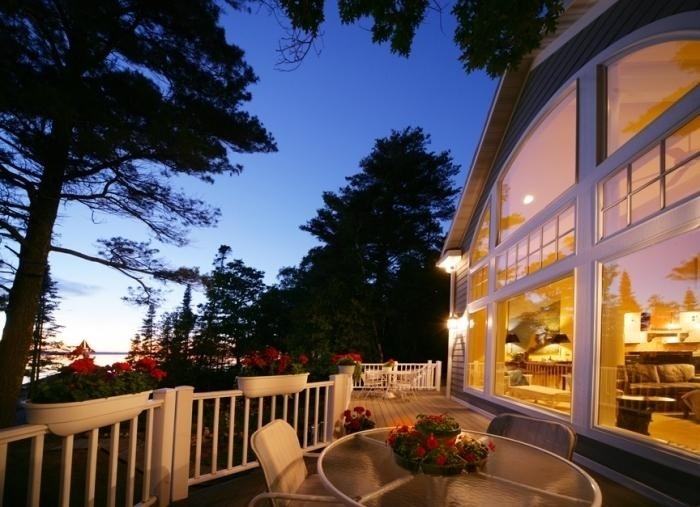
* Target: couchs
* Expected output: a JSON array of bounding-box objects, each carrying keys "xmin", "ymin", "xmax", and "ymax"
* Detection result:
[{"xmin": 618, "ymin": 360, "xmax": 700, "ymax": 409}]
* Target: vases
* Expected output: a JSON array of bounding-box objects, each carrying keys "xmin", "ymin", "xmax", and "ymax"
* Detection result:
[
  {"xmin": 338, "ymin": 365, "xmax": 354, "ymax": 371},
  {"xmin": 236, "ymin": 373, "xmax": 310, "ymax": 399},
  {"xmin": 395, "ymin": 459, "xmax": 466, "ymax": 474},
  {"xmin": 18, "ymin": 388, "xmax": 155, "ymax": 438}
]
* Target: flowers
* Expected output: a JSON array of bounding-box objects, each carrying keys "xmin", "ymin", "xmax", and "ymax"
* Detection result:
[
  {"xmin": 232, "ymin": 347, "xmax": 310, "ymax": 374},
  {"xmin": 19, "ymin": 351, "xmax": 169, "ymax": 401},
  {"xmin": 327, "ymin": 350, "xmax": 364, "ymax": 363},
  {"xmin": 341, "ymin": 404, "xmax": 375, "ymax": 431},
  {"xmin": 386, "ymin": 409, "xmax": 497, "ymax": 462}
]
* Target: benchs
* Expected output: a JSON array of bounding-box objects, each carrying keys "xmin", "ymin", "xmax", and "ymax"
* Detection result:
[{"xmin": 508, "ymin": 382, "xmax": 566, "ymax": 406}]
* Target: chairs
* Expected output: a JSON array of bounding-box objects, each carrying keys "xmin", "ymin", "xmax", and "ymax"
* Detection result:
[
  {"xmin": 246, "ymin": 418, "xmax": 335, "ymax": 506},
  {"xmin": 359, "ymin": 366, "xmax": 426, "ymax": 402},
  {"xmin": 503, "ymin": 365, "xmax": 533, "ymax": 395},
  {"xmin": 487, "ymin": 411, "xmax": 578, "ymax": 462}
]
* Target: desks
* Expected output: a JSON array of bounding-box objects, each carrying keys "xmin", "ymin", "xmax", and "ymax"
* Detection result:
[{"xmin": 313, "ymin": 424, "xmax": 605, "ymax": 507}]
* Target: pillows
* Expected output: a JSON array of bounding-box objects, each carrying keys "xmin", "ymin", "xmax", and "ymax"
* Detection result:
[{"xmin": 510, "ymin": 370, "xmax": 527, "ymax": 387}]
